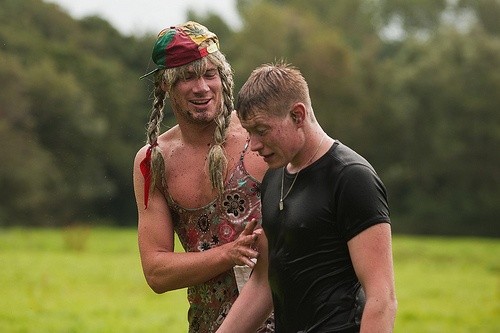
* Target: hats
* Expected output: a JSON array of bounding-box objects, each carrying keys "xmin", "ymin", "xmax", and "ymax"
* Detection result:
[{"xmin": 138, "ymin": 20, "xmax": 219, "ymax": 80}]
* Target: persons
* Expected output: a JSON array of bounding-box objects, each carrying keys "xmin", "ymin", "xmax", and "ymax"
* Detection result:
[
  {"xmin": 133, "ymin": 19, "xmax": 276, "ymax": 333},
  {"xmin": 213, "ymin": 57, "xmax": 397, "ymax": 333}
]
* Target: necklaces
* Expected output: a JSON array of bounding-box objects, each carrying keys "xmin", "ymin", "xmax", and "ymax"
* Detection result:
[{"xmin": 279, "ymin": 131, "xmax": 327, "ymax": 211}]
